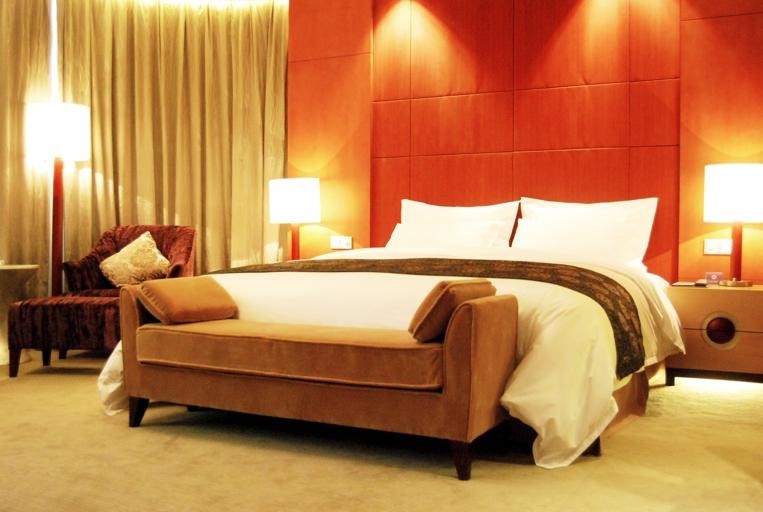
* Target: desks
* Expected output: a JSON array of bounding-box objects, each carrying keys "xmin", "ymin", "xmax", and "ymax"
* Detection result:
[{"xmin": 0, "ymin": 264, "xmax": 44, "ymax": 365}]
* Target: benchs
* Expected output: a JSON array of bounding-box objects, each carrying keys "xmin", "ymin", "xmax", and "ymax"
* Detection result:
[{"xmin": 118, "ymin": 279, "xmax": 520, "ymax": 480}]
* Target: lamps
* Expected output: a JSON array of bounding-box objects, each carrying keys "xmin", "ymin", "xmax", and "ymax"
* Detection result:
[
  {"xmin": 267, "ymin": 176, "xmax": 322, "ymax": 261},
  {"xmin": 702, "ymin": 162, "xmax": 763, "ymax": 283},
  {"xmin": 29, "ymin": 102, "xmax": 90, "ymax": 298}
]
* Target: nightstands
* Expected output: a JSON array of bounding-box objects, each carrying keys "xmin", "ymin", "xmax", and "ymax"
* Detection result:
[{"xmin": 669, "ymin": 278, "xmax": 762, "ymax": 384}]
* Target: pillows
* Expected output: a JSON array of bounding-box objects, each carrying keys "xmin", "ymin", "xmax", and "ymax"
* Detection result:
[
  {"xmin": 96, "ymin": 231, "xmax": 170, "ymax": 291},
  {"xmin": 399, "ymin": 198, "xmax": 520, "ymax": 261},
  {"xmin": 511, "ymin": 197, "xmax": 659, "ymax": 275}
]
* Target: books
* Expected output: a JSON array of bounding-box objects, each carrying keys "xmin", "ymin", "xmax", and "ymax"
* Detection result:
[{"xmin": 672, "ymin": 282, "xmax": 695, "ymax": 286}]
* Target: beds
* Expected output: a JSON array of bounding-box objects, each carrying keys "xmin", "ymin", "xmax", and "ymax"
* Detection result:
[{"xmin": 99, "ymin": 196, "xmax": 688, "ymax": 470}]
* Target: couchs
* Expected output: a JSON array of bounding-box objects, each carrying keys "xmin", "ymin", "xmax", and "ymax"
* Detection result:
[{"xmin": 8, "ymin": 225, "xmax": 196, "ymax": 381}]
